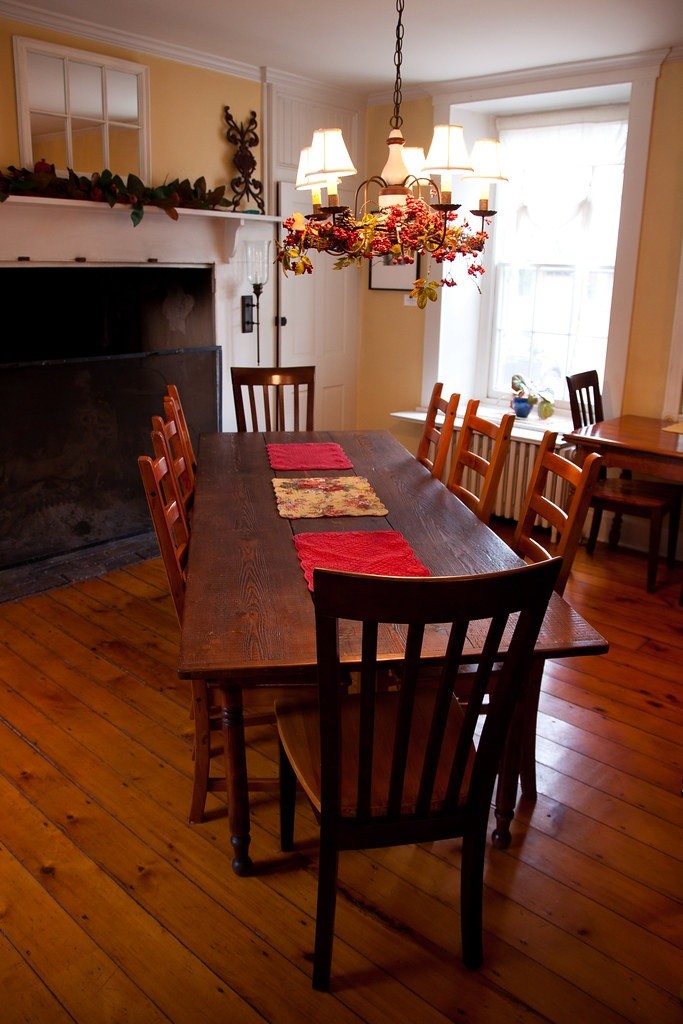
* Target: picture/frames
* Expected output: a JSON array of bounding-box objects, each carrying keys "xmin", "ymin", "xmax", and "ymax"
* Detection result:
[{"xmin": 368, "ymin": 248, "xmax": 420, "ymax": 292}]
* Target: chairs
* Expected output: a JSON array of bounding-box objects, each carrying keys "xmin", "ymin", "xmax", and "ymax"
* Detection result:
[{"xmin": 137, "ymin": 364, "xmax": 683, "ymax": 991}]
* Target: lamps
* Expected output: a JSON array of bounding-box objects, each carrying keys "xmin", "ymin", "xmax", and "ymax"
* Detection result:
[{"xmin": 275, "ymin": 0, "xmax": 511, "ymax": 304}]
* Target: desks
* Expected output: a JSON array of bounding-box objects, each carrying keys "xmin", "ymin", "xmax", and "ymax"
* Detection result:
[
  {"xmin": 176, "ymin": 427, "xmax": 611, "ymax": 878},
  {"xmin": 563, "ymin": 415, "xmax": 683, "ymax": 518}
]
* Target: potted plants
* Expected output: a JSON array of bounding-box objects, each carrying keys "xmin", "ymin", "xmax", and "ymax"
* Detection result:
[{"xmin": 511, "ymin": 375, "xmax": 554, "ymax": 420}]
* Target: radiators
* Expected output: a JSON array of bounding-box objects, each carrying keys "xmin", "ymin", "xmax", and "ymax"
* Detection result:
[{"xmin": 430, "ymin": 427, "xmax": 578, "ymax": 545}]
